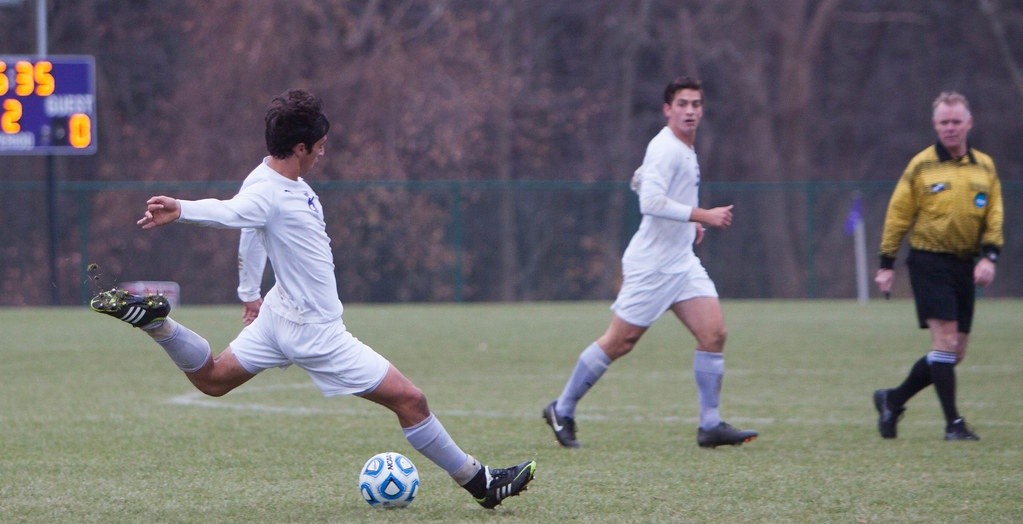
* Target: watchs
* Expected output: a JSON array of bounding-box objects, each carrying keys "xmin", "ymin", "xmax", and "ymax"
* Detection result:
[{"xmin": 984, "ymin": 252, "xmax": 998, "ymax": 262}]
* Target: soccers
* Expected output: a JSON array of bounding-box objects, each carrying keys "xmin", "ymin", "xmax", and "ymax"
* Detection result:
[{"xmin": 359, "ymin": 452, "xmax": 420, "ymax": 509}]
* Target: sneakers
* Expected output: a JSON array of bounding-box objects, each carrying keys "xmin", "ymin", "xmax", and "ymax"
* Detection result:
[
  {"xmin": 697, "ymin": 422, "xmax": 759, "ymax": 448},
  {"xmin": 90, "ymin": 288, "xmax": 170, "ymax": 328},
  {"xmin": 873, "ymin": 388, "xmax": 906, "ymax": 439},
  {"xmin": 943, "ymin": 417, "xmax": 980, "ymax": 440},
  {"xmin": 542, "ymin": 400, "xmax": 578, "ymax": 447},
  {"xmin": 472, "ymin": 460, "xmax": 536, "ymax": 510}
]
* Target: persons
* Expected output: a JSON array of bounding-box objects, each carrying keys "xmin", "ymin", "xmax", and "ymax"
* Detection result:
[
  {"xmin": 874, "ymin": 93, "xmax": 1004, "ymax": 440},
  {"xmin": 544, "ymin": 77, "xmax": 757, "ymax": 449},
  {"xmin": 91, "ymin": 89, "xmax": 537, "ymax": 509}
]
